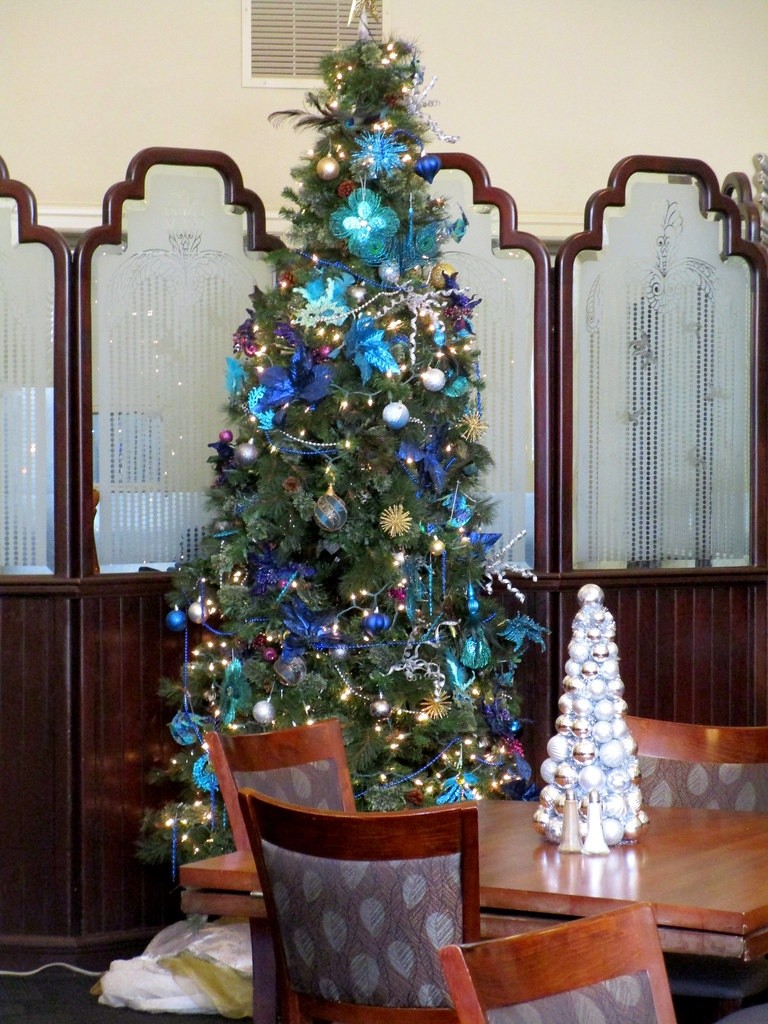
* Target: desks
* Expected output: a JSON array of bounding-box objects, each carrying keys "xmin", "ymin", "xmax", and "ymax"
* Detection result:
[{"xmin": 173, "ymin": 799, "xmax": 768, "ymax": 963}]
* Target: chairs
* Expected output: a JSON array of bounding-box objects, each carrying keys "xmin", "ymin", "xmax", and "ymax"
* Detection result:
[{"xmin": 204, "ymin": 714, "xmax": 768, "ymax": 1024}]
